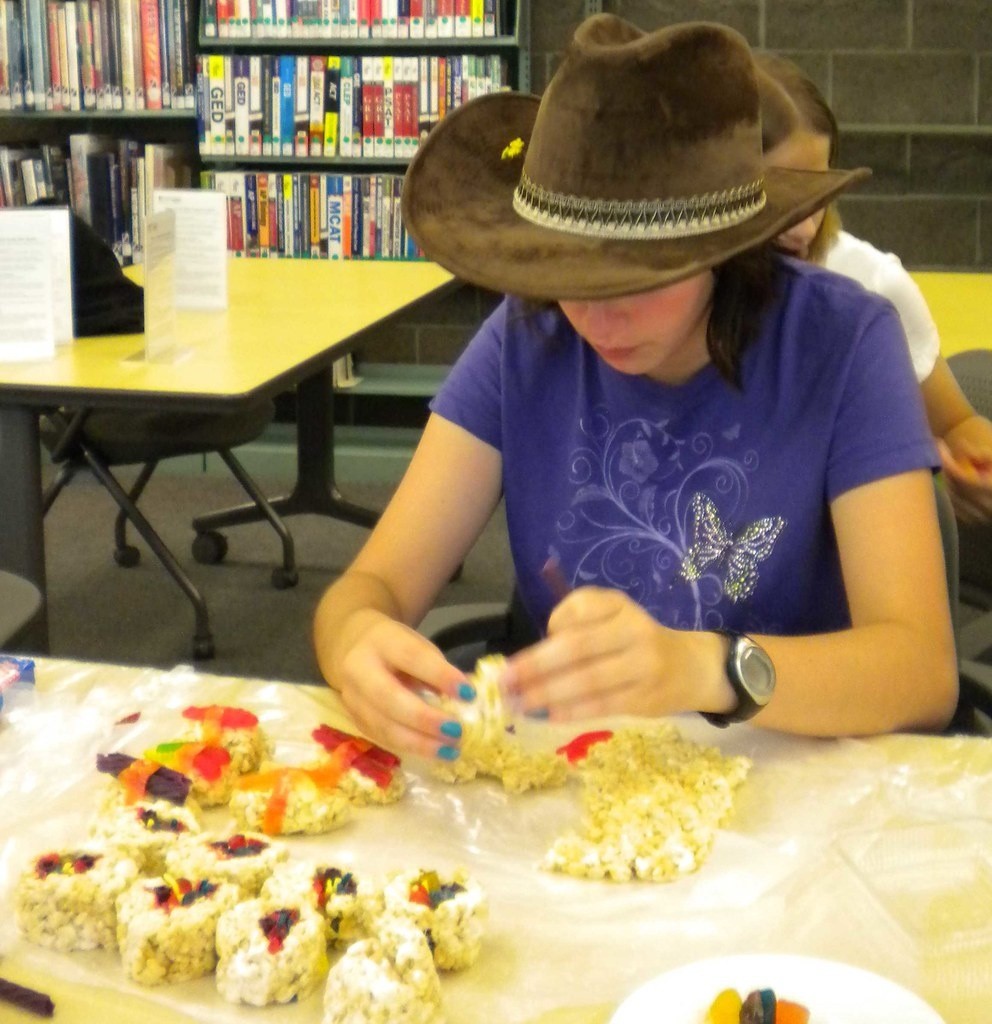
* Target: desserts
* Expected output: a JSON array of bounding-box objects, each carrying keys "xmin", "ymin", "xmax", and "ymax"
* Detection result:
[{"xmin": 21, "ymin": 651, "xmax": 751, "ymax": 1024}]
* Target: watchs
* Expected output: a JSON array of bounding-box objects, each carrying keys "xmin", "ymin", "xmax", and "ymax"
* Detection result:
[{"xmin": 698, "ymin": 625, "xmax": 776, "ymax": 730}]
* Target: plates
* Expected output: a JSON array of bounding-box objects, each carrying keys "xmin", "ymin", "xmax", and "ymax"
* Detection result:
[{"xmin": 608, "ymin": 952, "xmax": 947, "ymax": 1024}]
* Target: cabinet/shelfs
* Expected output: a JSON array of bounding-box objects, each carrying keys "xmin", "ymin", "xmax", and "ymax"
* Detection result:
[{"xmin": 1, "ymin": 3, "xmax": 528, "ymax": 394}]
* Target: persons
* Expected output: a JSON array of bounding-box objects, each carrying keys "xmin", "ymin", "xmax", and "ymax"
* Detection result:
[
  {"xmin": 309, "ymin": 13, "xmax": 961, "ymax": 765},
  {"xmin": 751, "ymin": 49, "xmax": 991, "ymax": 532}
]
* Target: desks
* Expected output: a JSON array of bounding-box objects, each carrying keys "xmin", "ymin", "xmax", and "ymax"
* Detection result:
[
  {"xmin": 0, "ymin": 655, "xmax": 992, "ymax": 1024},
  {"xmin": 0, "ymin": 253, "xmax": 464, "ymax": 644}
]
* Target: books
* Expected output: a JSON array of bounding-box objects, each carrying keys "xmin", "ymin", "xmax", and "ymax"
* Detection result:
[
  {"xmin": 194, "ymin": 53, "xmax": 512, "ymax": 157},
  {"xmin": 0, "ymin": 127, "xmax": 193, "ymax": 268},
  {"xmin": 196, "ymin": 169, "xmax": 432, "ymax": 261},
  {"xmin": 0, "ymin": 1, "xmax": 195, "ymax": 109},
  {"xmin": 198, "ymin": 0, "xmax": 509, "ymax": 38}
]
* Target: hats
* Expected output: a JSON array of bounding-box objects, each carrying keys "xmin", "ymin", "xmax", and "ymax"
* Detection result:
[{"xmin": 395, "ymin": 9, "xmax": 877, "ymax": 316}]
